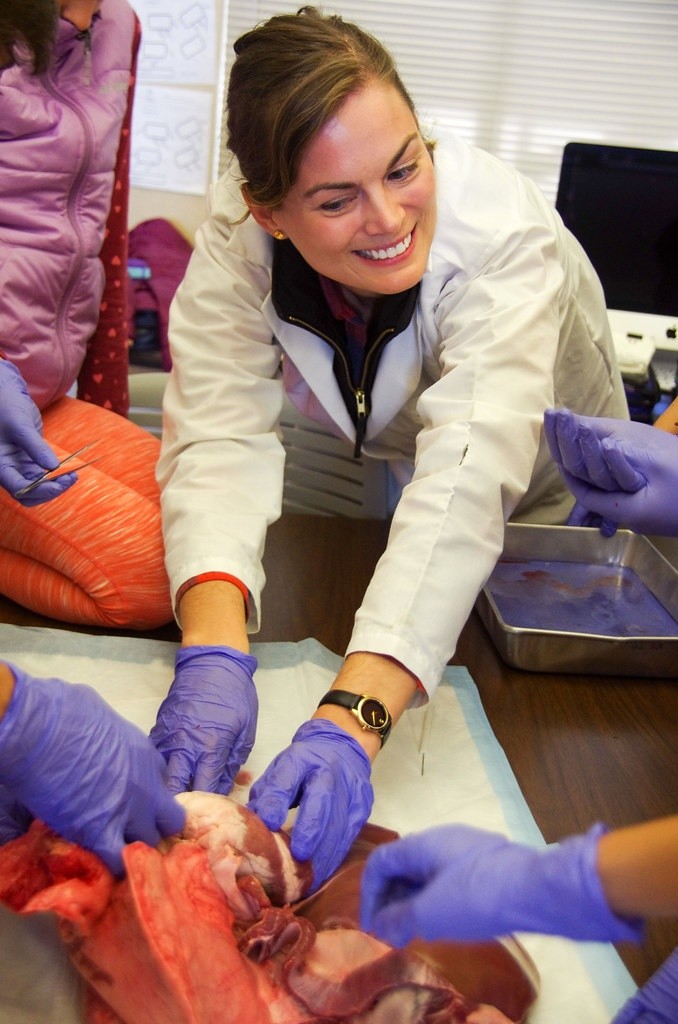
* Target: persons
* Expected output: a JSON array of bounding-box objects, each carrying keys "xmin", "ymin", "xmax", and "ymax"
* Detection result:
[
  {"xmin": 141, "ymin": 5, "xmax": 634, "ymax": 891},
  {"xmin": 0, "ymin": 660, "xmax": 189, "ymax": 878},
  {"xmin": 544, "ymin": 407, "xmax": 678, "ymax": 538},
  {"xmin": 361, "ymin": 813, "xmax": 678, "ymax": 1024},
  {"xmin": 0, "ymin": 1, "xmax": 175, "ymax": 630}
]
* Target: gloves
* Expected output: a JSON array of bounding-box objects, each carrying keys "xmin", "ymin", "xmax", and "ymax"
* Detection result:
[
  {"xmin": 247, "ymin": 716, "xmax": 378, "ymax": 894},
  {"xmin": 360, "ymin": 819, "xmax": 642, "ymax": 951},
  {"xmin": 143, "ymin": 643, "xmax": 260, "ymax": 799},
  {"xmin": 0, "ymin": 350, "xmax": 79, "ymax": 508},
  {"xmin": 543, "ymin": 405, "xmax": 678, "ymax": 539},
  {"xmin": 1, "ymin": 658, "xmax": 188, "ymax": 878},
  {"xmin": 611, "ymin": 947, "xmax": 678, "ymax": 1024}
]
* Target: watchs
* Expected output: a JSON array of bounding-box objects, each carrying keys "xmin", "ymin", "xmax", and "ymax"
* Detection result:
[{"xmin": 317, "ymin": 690, "xmax": 393, "ymax": 751}]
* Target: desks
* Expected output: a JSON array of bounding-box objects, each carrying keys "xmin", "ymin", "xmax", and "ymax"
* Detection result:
[{"xmin": 0, "ymin": 515, "xmax": 678, "ymax": 985}]
